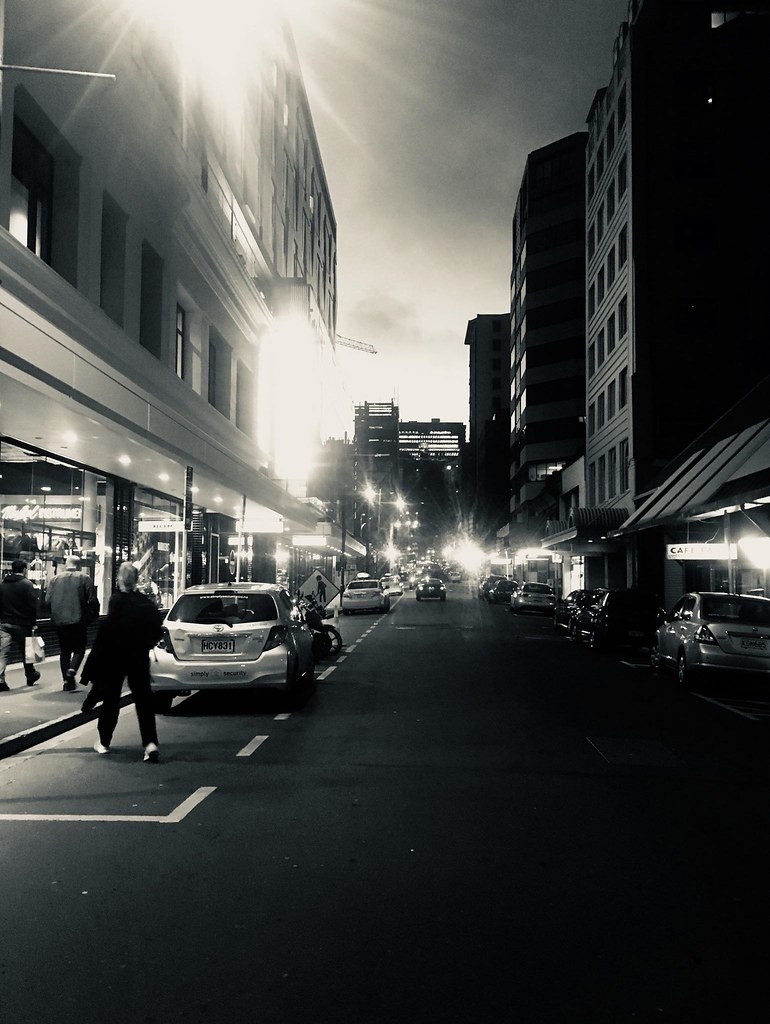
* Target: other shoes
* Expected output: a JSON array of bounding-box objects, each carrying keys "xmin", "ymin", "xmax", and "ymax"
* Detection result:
[
  {"xmin": 26, "ymin": 671, "xmax": 40, "ymax": 686},
  {"xmin": 66, "ymin": 669, "xmax": 76, "ymax": 686},
  {"xmin": 63, "ymin": 683, "xmax": 75, "ymax": 691},
  {"xmin": 0, "ymin": 683, "xmax": 11, "ymax": 692}
]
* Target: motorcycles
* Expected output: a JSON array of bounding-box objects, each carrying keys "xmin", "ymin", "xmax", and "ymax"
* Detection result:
[{"xmin": 295, "ymin": 588, "xmax": 342, "ymax": 656}]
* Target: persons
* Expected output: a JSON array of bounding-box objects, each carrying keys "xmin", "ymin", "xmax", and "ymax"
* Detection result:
[
  {"xmin": 0, "ymin": 560, "xmax": 41, "ymax": 692},
  {"xmin": 93, "ymin": 561, "xmax": 163, "ymax": 760},
  {"xmin": 45, "ymin": 554, "xmax": 99, "ymax": 690}
]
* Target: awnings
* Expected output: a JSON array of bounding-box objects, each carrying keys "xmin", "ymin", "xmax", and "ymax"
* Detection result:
[{"xmin": 601, "ymin": 418, "xmax": 770, "ymax": 540}]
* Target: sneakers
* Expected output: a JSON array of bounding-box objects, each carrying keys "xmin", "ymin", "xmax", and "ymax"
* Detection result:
[
  {"xmin": 94, "ymin": 741, "xmax": 110, "ymax": 753},
  {"xmin": 144, "ymin": 742, "xmax": 158, "ymax": 761}
]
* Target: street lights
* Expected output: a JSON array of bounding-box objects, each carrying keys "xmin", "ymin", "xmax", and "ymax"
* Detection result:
[{"xmin": 339, "ymin": 452, "xmax": 391, "ymax": 604}]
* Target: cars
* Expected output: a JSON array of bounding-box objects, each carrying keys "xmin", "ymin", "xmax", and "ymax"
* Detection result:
[
  {"xmin": 340, "ymin": 579, "xmax": 390, "ymax": 616},
  {"xmin": 476, "ymin": 573, "xmax": 518, "ymax": 603},
  {"xmin": 147, "ymin": 581, "xmax": 322, "ymax": 714},
  {"xmin": 510, "ymin": 581, "xmax": 558, "ymax": 617},
  {"xmin": 399, "ymin": 560, "xmax": 462, "ymax": 591},
  {"xmin": 552, "ymin": 587, "xmax": 667, "ymax": 658},
  {"xmin": 648, "ymin": 591, "xmax": 770, "ymax": 689},
  {"xmin": 379, "ymin": 576, "xmax": 404, "ymax": 597},
  {"xmin": 416, "ymin": 577, "xmax": 447, "ymax": 601}
]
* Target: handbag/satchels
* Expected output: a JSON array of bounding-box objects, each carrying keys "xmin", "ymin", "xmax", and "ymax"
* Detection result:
[
  {"xmin": 25, "ymin": 629, "xmax": 45, "ymax": 664},
  {"xmin": 77, "ymin": 575, "xmax": 99, "ymax": 625}
]
